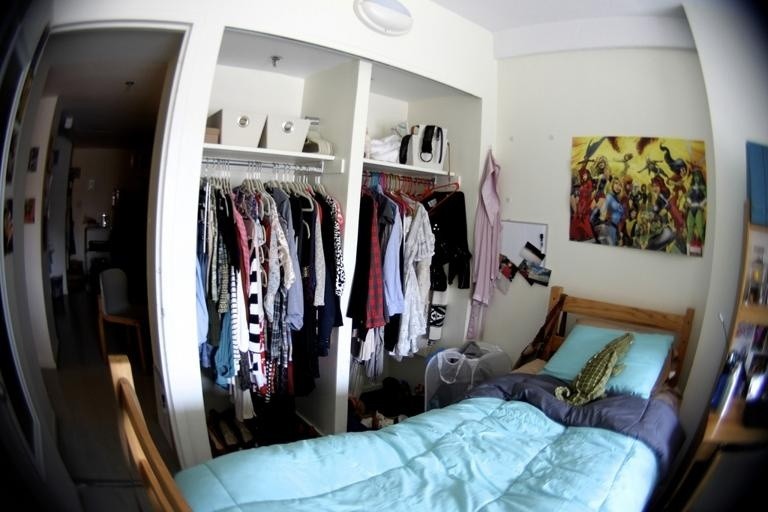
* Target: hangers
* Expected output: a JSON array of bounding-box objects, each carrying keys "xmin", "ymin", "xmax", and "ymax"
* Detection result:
[
  {"xmin": 359, "ymin": 168, "xmax": 459, "ymax": 217},
  {"xmin": 203, "ymin": 159, "xmax": 326, "ymax": 253}
]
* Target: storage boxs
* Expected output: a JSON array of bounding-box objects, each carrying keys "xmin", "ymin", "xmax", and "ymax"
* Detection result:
[
  {"xmin": 402, "ymin": 123, "xmax": 448, "ymax": 171},
  {"xmin": 207, "ymin": 108, "xmax": 311, "ymax": 154}
]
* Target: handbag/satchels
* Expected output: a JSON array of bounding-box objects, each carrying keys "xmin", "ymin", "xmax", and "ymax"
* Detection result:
[{"xmin": 513, "ymin": 293, "xmax": 570, "ymax": 369}]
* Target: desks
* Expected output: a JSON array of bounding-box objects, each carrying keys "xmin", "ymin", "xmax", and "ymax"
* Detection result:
[{"xmin": 663, "ymin": 396, "xmax": 768, "ymax": 512}]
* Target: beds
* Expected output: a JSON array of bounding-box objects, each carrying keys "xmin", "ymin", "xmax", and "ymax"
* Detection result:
[{"xmin": 106, "ymin": 285, "xmax": 695, "ymax": 512}]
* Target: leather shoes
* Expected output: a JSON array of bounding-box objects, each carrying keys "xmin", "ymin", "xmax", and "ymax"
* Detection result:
[{"xmin": 206, "ymin": 409, "xmax": 260, "ymax": 457}]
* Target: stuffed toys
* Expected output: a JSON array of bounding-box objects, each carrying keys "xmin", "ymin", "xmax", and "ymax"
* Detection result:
[{"xmin": 554, "ymin": 332, "xmax": 634, "ymax": 407}]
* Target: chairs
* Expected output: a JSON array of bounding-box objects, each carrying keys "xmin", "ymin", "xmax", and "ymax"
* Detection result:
[{"xmin": 98, "ymin": 268, "xmax": 149, "ymax": 373}]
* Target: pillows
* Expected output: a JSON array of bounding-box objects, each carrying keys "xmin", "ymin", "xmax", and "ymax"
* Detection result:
[{"xmin": 533, "ymin": 323, "xmax": 675, "ymax": 399}]
